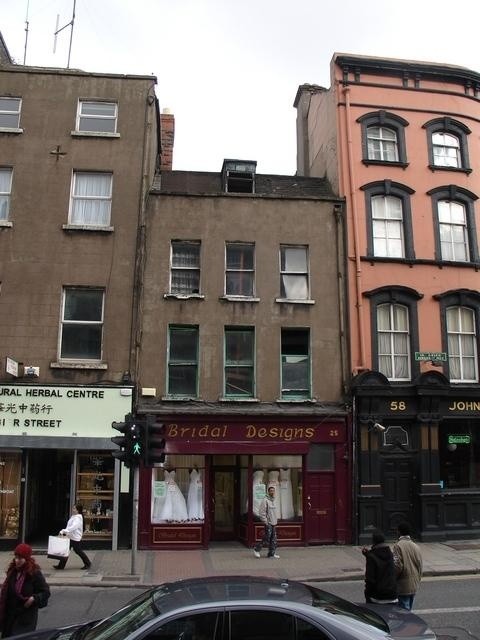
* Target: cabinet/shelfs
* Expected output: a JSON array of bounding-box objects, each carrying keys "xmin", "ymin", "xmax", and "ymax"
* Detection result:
[{"xmin": 76, "ymin": 453, "xmax": 115, "ymax": 539}]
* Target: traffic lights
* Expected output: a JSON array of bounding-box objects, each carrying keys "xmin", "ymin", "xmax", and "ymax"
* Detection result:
[
  {"xmin": 144, "ymin": 414, "xmax": 167, "ymax": 467},
  {"xmin": 124, "ymin": 420, "xmax": 148, "ymax": 466},
  {"xmin": 110, "ymin": 413, "xmax": 134, "ymax": 469}
]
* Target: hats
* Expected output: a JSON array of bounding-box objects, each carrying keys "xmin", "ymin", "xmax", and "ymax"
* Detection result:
[{"xmin": 14, "ymin": 544, "xmax": 32, "ymax": 559}]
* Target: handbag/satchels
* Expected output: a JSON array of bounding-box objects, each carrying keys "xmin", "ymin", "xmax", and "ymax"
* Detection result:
[{"xmin": 47, "ymin": 533, "xmax": 70, "ymax": 557}]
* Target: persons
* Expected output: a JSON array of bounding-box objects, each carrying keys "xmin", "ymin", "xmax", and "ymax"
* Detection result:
[
  {"xmin": 266, "ymin": 467, "xmax": 280, "ymax": 521},
  {"xmin": 153, "ymin": 466, "xmax": 189, "ymax": 523},
  {"xmin": 186, "ymin": 464, "xmax": 206, "ymax": 521},
  {"xmin": 280, "ymin": 468, "xmax": 295, "ymax": 520},
  {"xmin": 245, "ymin": 464, "xmax": 266, "ymax": 518},
  {"xmin": 0, "ymin": 543, "xmax": 50, "ymax": 639},
  {"xmin": 52, "ymin": 503, "xmax": 92, "ymax": 570},
  {"xmin": 361, "ymin": 527, "xmax": 398, "ymax": 608},
  {"xmin": 394, "ymin": 522, "xmax": 424, "ymax": 611},
  {"xmin": 251, "ymin": 486, "xmax": 281, "ymax": 560}
]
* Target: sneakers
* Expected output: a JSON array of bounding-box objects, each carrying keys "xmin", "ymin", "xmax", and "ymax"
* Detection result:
[
  {"xmin": 269, "ymin": 554, "xmax": 280, "ymax": 559},
  {"xmin": 254, "ymin": 550, "xmax": 260, "ymax": 558}
]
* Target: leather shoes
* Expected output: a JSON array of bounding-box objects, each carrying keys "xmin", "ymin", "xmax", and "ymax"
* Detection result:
[
  {"xmin": 81, "ymin": 566, "xmax": 87, "ymax": 570},
  {"xmin": 53, "ymin": 566, "xmax": 64, "ymax": 569}
]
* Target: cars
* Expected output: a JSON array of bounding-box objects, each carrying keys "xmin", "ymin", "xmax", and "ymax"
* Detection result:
[{"xmin": 3, "ymin": 574, "xmax": 442, "ymax": 640}]
